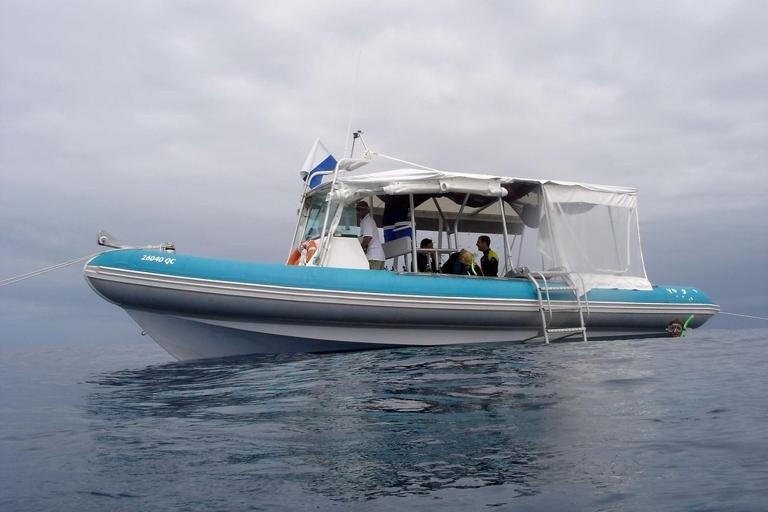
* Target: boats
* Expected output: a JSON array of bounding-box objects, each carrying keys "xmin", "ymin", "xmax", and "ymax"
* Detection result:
[{"xmin": 79, "ymin": 123, "xmax": 724, "ymax": 363}]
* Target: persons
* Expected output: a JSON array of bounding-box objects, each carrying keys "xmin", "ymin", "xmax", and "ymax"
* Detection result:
[
  {"xmin": 357, "ymin": 200, "xmax": 386, "ymax": 269},
  {"xmin": 411, "ymin": 238, "xmax": 436, "ymax": 272},
  {"xmin": 477, "ymin": 235, "xmax": 500, "ymax": 276},
  {"xmin": 460, "ymin": 250, "xmax": 483, "ymax": 276}
]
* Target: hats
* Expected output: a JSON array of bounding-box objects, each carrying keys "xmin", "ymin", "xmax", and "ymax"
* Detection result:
[{"xmin": 357, "ymin": 201, "xmax": 368, "ymax": 208}]
